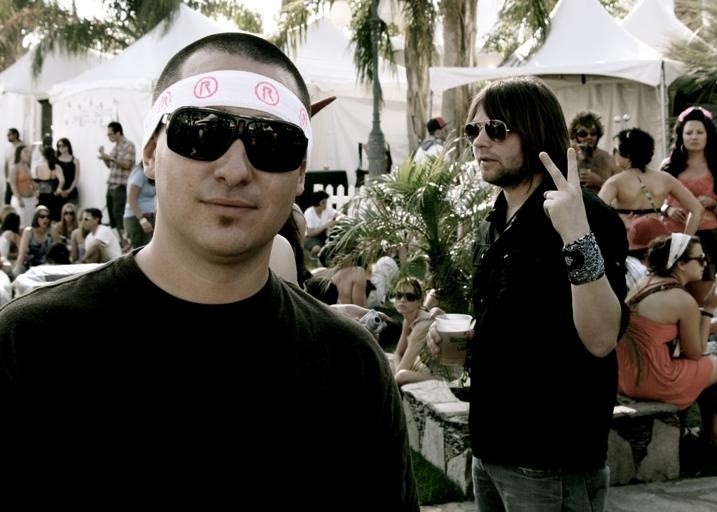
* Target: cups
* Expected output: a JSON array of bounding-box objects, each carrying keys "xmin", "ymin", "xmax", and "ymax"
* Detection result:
[
  {"xmin": 435, "ymin": 313, "xmax": 475, "ymax": 367},
  {"xmin": 578, "ymin": 167, "xmax": 591, "ymax": 186}
]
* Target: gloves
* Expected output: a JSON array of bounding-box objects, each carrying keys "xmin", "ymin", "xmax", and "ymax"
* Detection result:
[{"xmin": 139, "ymin": 217, "xmax": 153, "ymax": 234}]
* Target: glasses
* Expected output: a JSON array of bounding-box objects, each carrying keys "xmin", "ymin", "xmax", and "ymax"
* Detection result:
[
  {"xmin": 395, "ymin": 291, "xmax": 417, "ymax": 302},
  {"xmin": 686, "ymin": 252, "xmax": 706, "ymax": 266},
  {"xmin": 575, "ymin": 127, "xmax": 599, "ymax": 137},
  {"xmin": 463, "ymin": 119, "xmax": 513, "ymax": 146},
  {"xmin": 612, "ymin": 144, "xmax": 620, "ymax": 154},
  {"xmin": 160, "ymin": 106, "xmax": 308, "ymax": 173},
  {"xmin": 38, "ymin": 211, "xmax": 92, "ymax": 222}
]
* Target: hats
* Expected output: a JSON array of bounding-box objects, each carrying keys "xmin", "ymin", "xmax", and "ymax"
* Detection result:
[
  {"xmin": 427, "ymin": 117, "xmax": 450, "ymax": 135},
  {"xmin": 677, "ymin": 107, "xmax": 713, "ymax": 125},
  {"xmin": 628, "ymin": 216, "xmax": 672, "ymax": 251}
]
